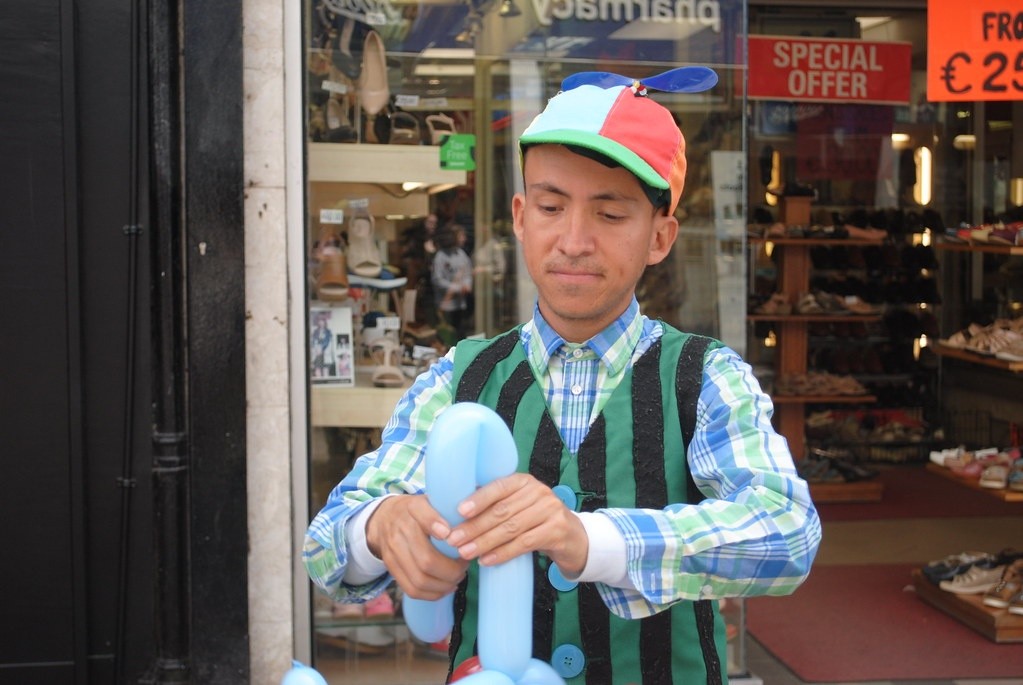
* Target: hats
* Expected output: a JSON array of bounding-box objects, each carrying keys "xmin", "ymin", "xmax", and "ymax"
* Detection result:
[{"xmin": 517, "ymin": 66, "xmax": 719, "ymax": 217}]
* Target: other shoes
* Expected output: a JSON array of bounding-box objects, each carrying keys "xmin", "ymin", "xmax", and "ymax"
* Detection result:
[
  {"xmin": 943, "ymin": 222, "xmax": 1023, "ymax": 245},
  {"xmin": 746, "ymin": 219, "xmax": 943, "ymax": 482},
  {"xmin": 315, "ymin": 590, "xmax": 396, "ymax": 617},
  {"xmin": 348, "ymin": 266, "xmax": 406, "ymax": 288}
]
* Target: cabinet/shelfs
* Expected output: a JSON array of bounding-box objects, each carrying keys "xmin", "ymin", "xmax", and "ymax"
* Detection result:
[
  {"xmin": 927, "ymin": 241, "xmax": 1023, "ymax": 502},
  {"xmin": 307, "ymin": 143, "xmax": 465, "ymax": 627},
  {"xmin": 748, "ymin": 196, "xmax": 888, "ymax": 503}
]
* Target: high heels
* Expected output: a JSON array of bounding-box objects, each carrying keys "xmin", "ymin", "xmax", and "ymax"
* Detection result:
[{"xmin": 326, "ymin": 8, "xmax": 459, "ymax": 146}]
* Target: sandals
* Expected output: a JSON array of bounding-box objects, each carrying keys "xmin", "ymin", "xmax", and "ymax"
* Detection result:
[
  {"xmin": 929, "ymin": 445, "xmax": 1023, "ymax": 490},
  {"xmin": 937, "ymin": 317, "xmax": 1023, "ymax": 360},
  {"xmin": 370, "ymin": 341, "xmax": 405, "ymax": 386},
  {"xmin": 315, "ymin": 232, "xmax": 350, "ymax": 301},
  {"xmin": 921, "ymin": 548, "xmax": 1023, "ymax": 613},
  {"xmin": 402, "ymin": 352, "xmax": 441, "ymax": 379},
  {"xmin": 346, "ymin": 208, "xmax": 383, "ymax": 279}
]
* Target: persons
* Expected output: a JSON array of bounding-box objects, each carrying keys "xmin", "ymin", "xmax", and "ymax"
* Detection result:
[
  {"xmin": 414, "ymin": 215, "xmax": 507, "ymax": 338},
  {"xmin": 303, "ymin": 65, "xmax": 823, "ymax": 685},
  {"xmin": 313, "ymin": 317, "xmax": 351, "ymax": 377}
]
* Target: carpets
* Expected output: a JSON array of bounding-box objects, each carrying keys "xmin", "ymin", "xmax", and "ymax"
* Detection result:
[{"xmin": 743, "ymin": 563, "xmax": 1023, "ymax": 685}]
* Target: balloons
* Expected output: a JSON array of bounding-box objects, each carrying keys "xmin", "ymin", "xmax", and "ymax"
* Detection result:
[
  {"xmin": 402, "ymin": 403, "xmax": 563, "ymax": 685},
  {"xmin": 283, "ymin": 662, "xmax": 326, "ymax": 685}
]
